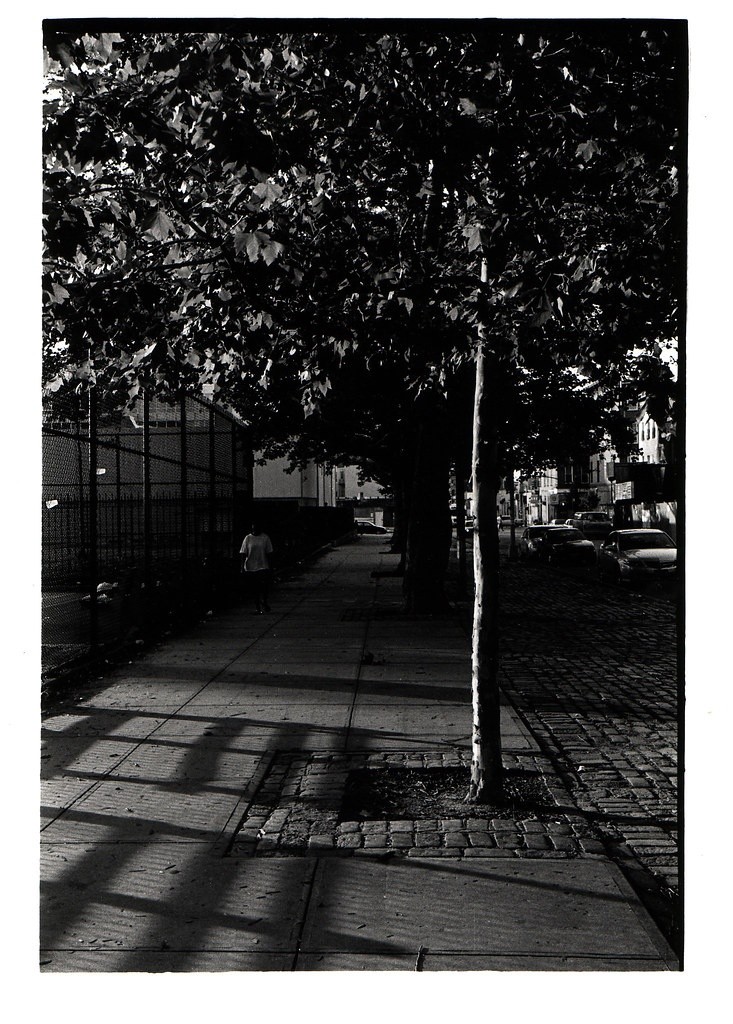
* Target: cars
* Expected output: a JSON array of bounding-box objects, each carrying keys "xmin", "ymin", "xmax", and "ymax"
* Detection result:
[
  {"xmin": 599, "ymin": 528, "xmax": 678, "ymax": 586},
  {"xmin": 538, "ymin": 527, "xmax": 597, "ymax": 568},
  {"xmin": 357, "ymin": 521, "xmax": 387, "ymax": 534},
  {"xmin": 547, "ymin": 518, "xmax": 574, "ymax": 528},
  {"xmin": 519, "ymin": 526, "xmax": 566, "ymax": 562},
  {"xmin": 463, "ymin": 514, "xmax": 524, "ymax": 533}
]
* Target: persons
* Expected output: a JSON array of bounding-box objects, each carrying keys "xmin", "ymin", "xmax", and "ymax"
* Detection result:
[
  {"xmin": 355, "ymin": 520, "xmax": 358, "ymax": 536},
  {"xmin": 497, "ymin": 515, "xmax": 504, "ymax": 531},
  {"xmin": 238, "ymin": 521, "xmax": 273, "ymax": 614},
  {"xmin": 472, "ymin": 515, "xmax": 477, "ymax": 520}
]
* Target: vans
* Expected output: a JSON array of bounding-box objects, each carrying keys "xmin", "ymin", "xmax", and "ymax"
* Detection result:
[{"xmin": 572, "ymin": 511, "xmax": 613, "ymax": 539}]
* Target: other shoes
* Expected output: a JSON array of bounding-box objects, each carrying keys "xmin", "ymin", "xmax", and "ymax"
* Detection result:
[
  {"xmin": 255, "ymin": 606, "xmax": 261, "ymax": 615},
  {"xmin": 265, "ymin": 604, "xmax": 271, "ymax": 612}
]
